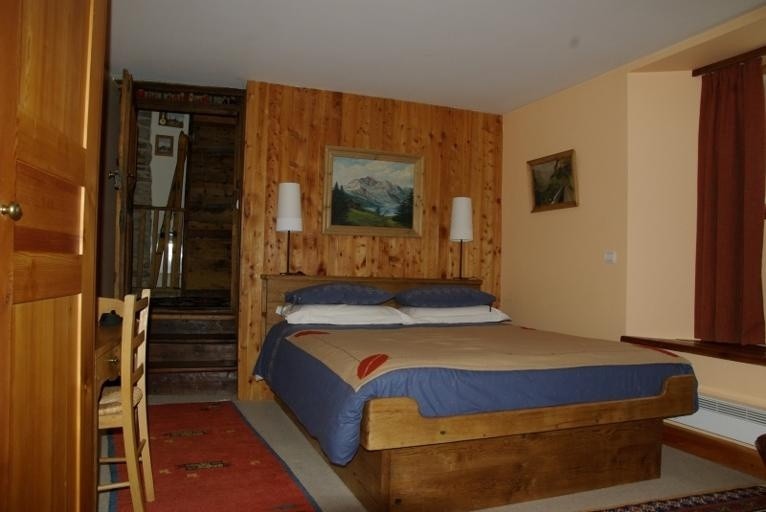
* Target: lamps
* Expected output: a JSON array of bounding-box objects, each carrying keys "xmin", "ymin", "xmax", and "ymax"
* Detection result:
[
  {"xmin": 276, "ymin": 182, "xmax": 305, "ymax": 276},
  {"xmin": 450, "ymin": 197, "xmax": 473, "ymax": 280}
]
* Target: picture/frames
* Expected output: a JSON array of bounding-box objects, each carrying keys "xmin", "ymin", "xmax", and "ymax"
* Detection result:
[
  {"xmin": 154, "ymin": 135, "xmax": 173, "ymax": 157},
  {"xmin": 527, "ymin": 149, "xmax": 579, "ymax": 213},
  {"xmin": 322, "ymin": 145, "xmax": 425, "ymax": 238}
]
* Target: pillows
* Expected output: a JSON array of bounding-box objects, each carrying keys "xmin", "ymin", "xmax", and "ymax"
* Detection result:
[{"xmin": 275, "ymin": 282, "xmax": 512, "ymax": 325}]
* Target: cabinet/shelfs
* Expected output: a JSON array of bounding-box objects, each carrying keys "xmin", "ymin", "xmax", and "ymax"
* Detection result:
[{"xmin": 0, "ymin": 0, "xmax": 113, "ymax": 512}]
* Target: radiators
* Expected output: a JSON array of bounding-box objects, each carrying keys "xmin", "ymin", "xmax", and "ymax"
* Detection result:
[{"xmin": 663, "ymin": 393, "xmax": 766, "ymax": 451}]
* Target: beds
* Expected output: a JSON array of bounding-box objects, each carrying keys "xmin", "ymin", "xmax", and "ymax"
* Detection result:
[{"xmin": 261, "ymin": 273, "xmax": 699, "ymax": 512}]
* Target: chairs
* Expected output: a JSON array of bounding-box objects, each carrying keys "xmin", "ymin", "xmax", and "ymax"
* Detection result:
[
  {"xmin": 96, "ymin": 288, "xmax": 155, "ymax": 512},
  {"xmin": 96, "ymin": 297, "xmax": 123, "ymax": 327}
]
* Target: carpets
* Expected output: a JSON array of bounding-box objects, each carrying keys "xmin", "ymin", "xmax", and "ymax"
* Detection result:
[
  {"xmin": 590, "ymin": 483, "xmax": 766, "ymax": 512},
  {"xmin": 107, "ymin": 399, "xmax": 322, "ymax": 512}
]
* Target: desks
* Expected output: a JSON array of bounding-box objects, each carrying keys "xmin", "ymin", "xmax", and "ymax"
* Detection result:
[
  {"xmin": 94, "ymin": 322, "xmax": 140, "ymax": 512},
  {"xmin": 620, "ymin": 335, "xmax": 766, "ymax": 451}
]
